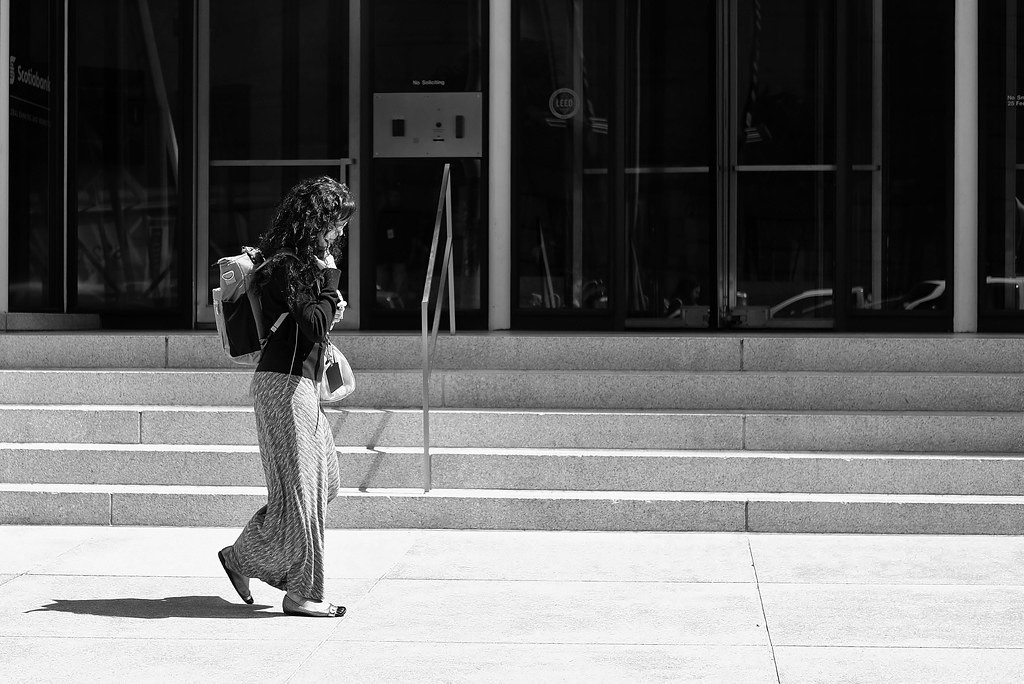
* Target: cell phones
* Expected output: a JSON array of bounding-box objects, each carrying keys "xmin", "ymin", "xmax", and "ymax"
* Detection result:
[{"xmin": 329, "ymin": 290, "xmax": 344, "ymax": 330}]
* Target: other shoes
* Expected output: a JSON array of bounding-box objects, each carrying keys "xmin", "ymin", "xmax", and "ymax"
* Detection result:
[
  {"xmin": 283, "ymin": 594, "xmax": 347, "ymax": 617},
  {"xmin": 218, "ymin": 546, "xmax": 254, "ymax": 604}
]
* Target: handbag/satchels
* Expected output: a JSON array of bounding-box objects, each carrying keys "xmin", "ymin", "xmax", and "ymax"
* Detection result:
[{"xmin": 316, "ymin": 340, "xmax": 355, "ymax": 403}]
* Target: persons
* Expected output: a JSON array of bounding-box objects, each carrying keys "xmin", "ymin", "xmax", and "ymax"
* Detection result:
[{"xmin": 218, "ymin": 178, "xmax": 352, "ymax": 619}]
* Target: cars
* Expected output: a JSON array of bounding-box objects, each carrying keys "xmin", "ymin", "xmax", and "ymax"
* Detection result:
[{"xmin": 769, "ymin": 275, "xmax": 1023, "ymax": 318}]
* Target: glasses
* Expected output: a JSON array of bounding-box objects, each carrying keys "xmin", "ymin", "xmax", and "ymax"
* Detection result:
[{"xmin": 334, "ymin": 222, "xmax": 348, "ymax": 231}]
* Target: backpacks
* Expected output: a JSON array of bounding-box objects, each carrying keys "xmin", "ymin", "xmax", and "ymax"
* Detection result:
[{"xmin": 210, "ymin": 246, "xmax": 321, "ymax": 365}]
